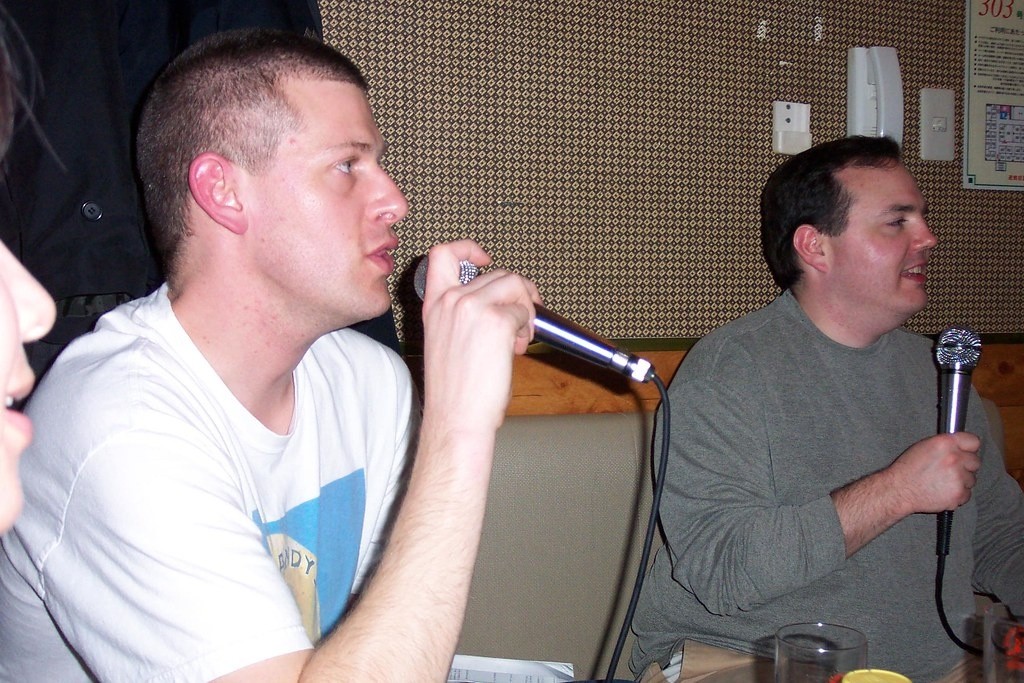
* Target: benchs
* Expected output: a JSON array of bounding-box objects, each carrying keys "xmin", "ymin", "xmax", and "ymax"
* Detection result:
[{"xmin": 396, "ymin": 338, "xmax": 1024, "ymax": 683}]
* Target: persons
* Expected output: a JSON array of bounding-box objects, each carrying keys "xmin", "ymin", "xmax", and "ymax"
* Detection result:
[
  {"xmin": 629, "ymin": 136, "xmax": 1024, "ymax": 683},
  {"xmin": 0, "ymin": 0, "xmax": 57, "ymax": 537},
  {"xmin": 0, "ymin": 28, "xmax": 544, "ymax": 683}
]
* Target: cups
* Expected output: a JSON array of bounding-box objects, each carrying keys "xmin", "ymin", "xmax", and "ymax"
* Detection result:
[
  {"xmin": 983, "ymin": 603, "xmax": 1024, "ymax": 683},
  {"xmin": 775, "ymin": 623, "xmax": 868, "ymax": 683},
  {"xmin": 841, "ymin": 669, "xmax": 911, "ymax": 683}
]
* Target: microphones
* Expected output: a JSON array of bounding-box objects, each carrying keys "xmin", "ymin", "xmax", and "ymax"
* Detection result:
[
  {"xmin": 935, "ymin": 326, "xmax": 983, "ymax": 554},
  {"xmin": 414, "ymin": 255, "xmax": 656, "ymax": 383}
]
